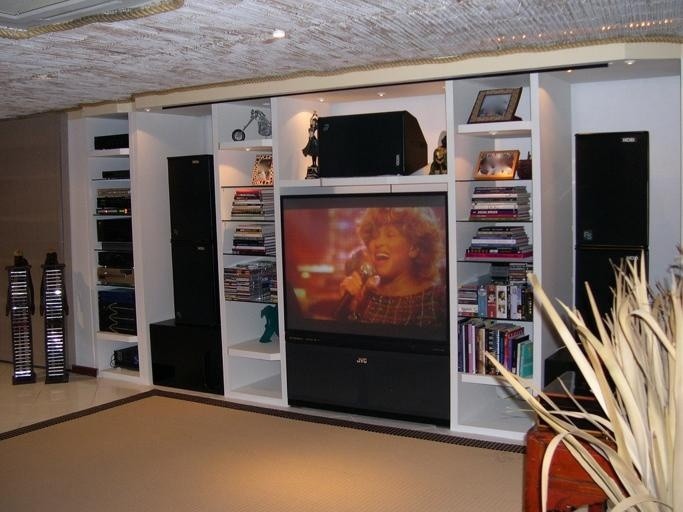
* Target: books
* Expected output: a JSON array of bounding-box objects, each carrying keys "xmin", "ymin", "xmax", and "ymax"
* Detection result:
[
  {"xmin": 456, "ymin": 180, "xmax": 532, "ymax": 379},
  {"xmin": 222, "ymin": 188, "xmax": 280, "ymax": 304}
]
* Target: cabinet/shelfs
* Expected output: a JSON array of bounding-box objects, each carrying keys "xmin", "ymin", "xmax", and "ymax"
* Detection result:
[
  {"xmin": 60, "ymin": 101, "xmax": 210, "ymax": 384},
  {"xmin": 441, "ymin": 70, "xmax": 573, "ymax": 444},
  {"xmin": 209, "ymin": 97, "xmax": 300, "ymax": 410}
]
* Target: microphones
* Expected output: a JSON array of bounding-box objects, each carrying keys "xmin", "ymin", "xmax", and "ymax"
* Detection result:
[{"xmin": 332, "ymin": 261, "xmax": 372, "ymax": 322}]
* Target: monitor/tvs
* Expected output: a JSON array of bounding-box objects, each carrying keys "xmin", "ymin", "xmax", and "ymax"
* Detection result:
[{"xmin": 278, "ymin": 192, "xmax": 446, "ymax": 348}]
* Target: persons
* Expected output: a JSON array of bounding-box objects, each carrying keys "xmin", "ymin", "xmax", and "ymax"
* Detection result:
[{"xmin": 330, "ymin": 206, "xmax": 449, "ymax": 330}]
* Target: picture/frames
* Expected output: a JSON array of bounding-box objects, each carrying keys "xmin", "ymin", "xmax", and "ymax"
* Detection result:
[
  {"xmin": 466, "ymin": 86, "xmax": 524, "ymax": 123},
  {"xmin": 472, "ymin": 147, "xmax": 519, "ymax": 179}
]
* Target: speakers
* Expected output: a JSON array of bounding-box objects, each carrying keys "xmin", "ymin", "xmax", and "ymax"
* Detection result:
[
  {"xmin": 574, "ymin": 132, "xmax": 651, "ymax": 389},
  {"xmin": 318, "ymin": 110, "xmax": 428, "ymax": 175},
  {"xmin": 166, "ymin": 152, "xmax": 224, "ymax": 330}
]
